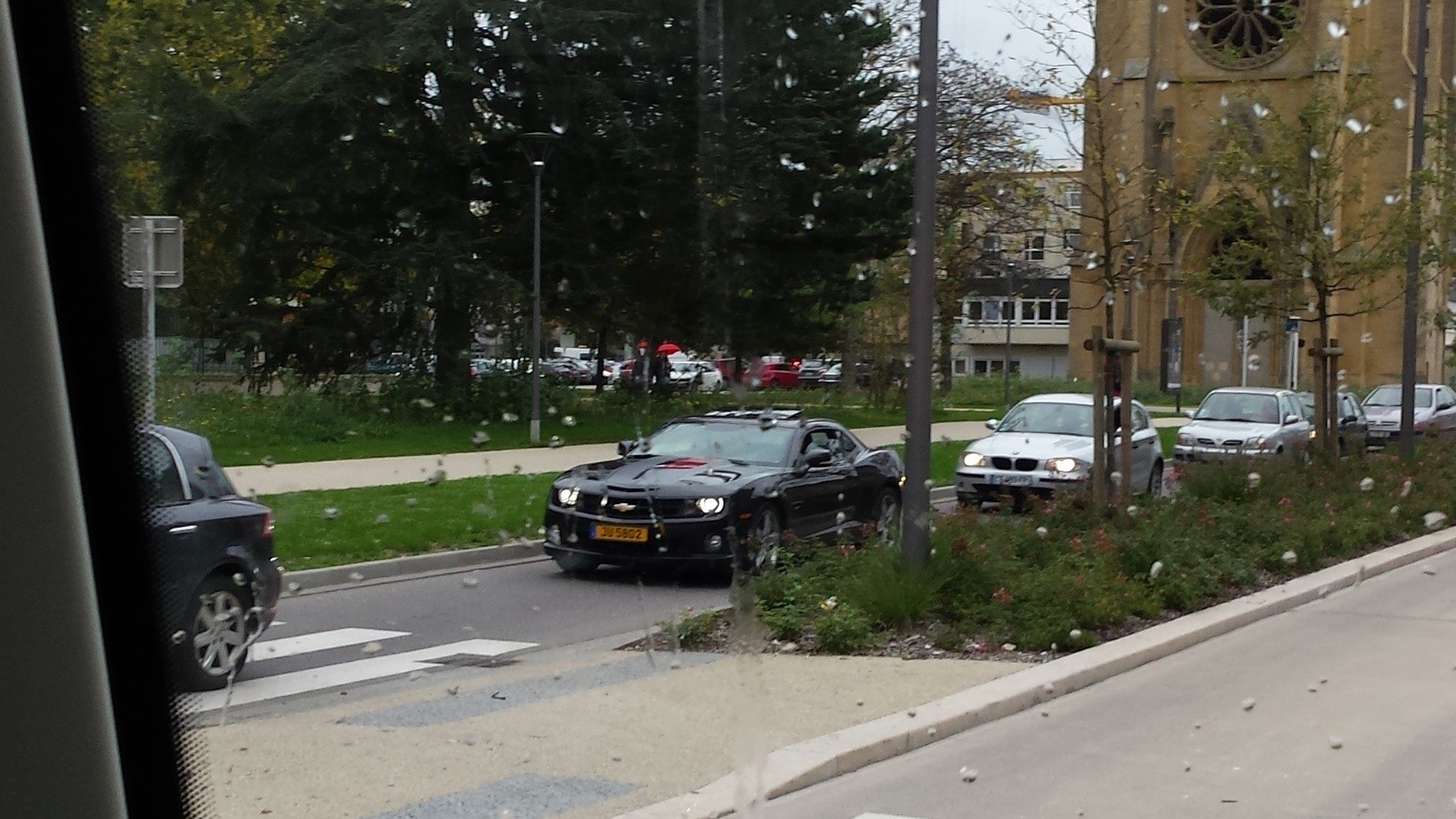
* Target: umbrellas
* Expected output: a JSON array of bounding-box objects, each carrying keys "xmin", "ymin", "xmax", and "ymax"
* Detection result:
[
  {"xmin": 656, "ymin": 343, "xmax": 679, "ymax": 357},
  {"xmin": 636, "ymin": 340, "xmax": 649, "ymax": 349}
]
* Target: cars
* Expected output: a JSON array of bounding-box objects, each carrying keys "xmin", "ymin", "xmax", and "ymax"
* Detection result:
[
  {"xmin": 797, "ymin": 359, "xmax": 842, "ymax": 386},
  {"xmin": 1360, "ymin": 384, "xmax": 1456, "ymax": 446},
  {"xmin": 386, "ymin": 346, "xmax": 754, "ymax": 398},
  {"xmin": 955, "ymin": 391, "xmax": 1165, "ymax": 518},
  {"xmin": 818, "ymin": 362, "xmax": 873, "ymax": 389},
  {"xmin": 541, "ymin": 407, "xmax": 904, "ymax": 581},
  {"xmin": 130, "ymin": 421, "xmax": 283, "ymax": 690},
  {"xmin": 1297, "ymin": 392, "xmax": 1371, "ymax": 461},
  {"xmin": 1173, "ymin": 387, "xmax": 1312, "ymax": 479},
  {"xmin": 753, "ymin": 363, "xmax": 800, "ymax": 389}
]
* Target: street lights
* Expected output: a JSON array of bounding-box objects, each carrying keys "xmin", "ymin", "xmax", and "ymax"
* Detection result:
[
  {"xmin": 514, "ymin": 133, "xmax": 563, "ymax": 445},
  {"xmin": 1005, "ymin": 262, "xmax": 1016, "ymax": 416}
]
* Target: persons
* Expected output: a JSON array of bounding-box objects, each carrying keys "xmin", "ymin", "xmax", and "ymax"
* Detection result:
[{"xmin": 632, "ymin": 350, "xmax": 672, "ymax": 400}]
